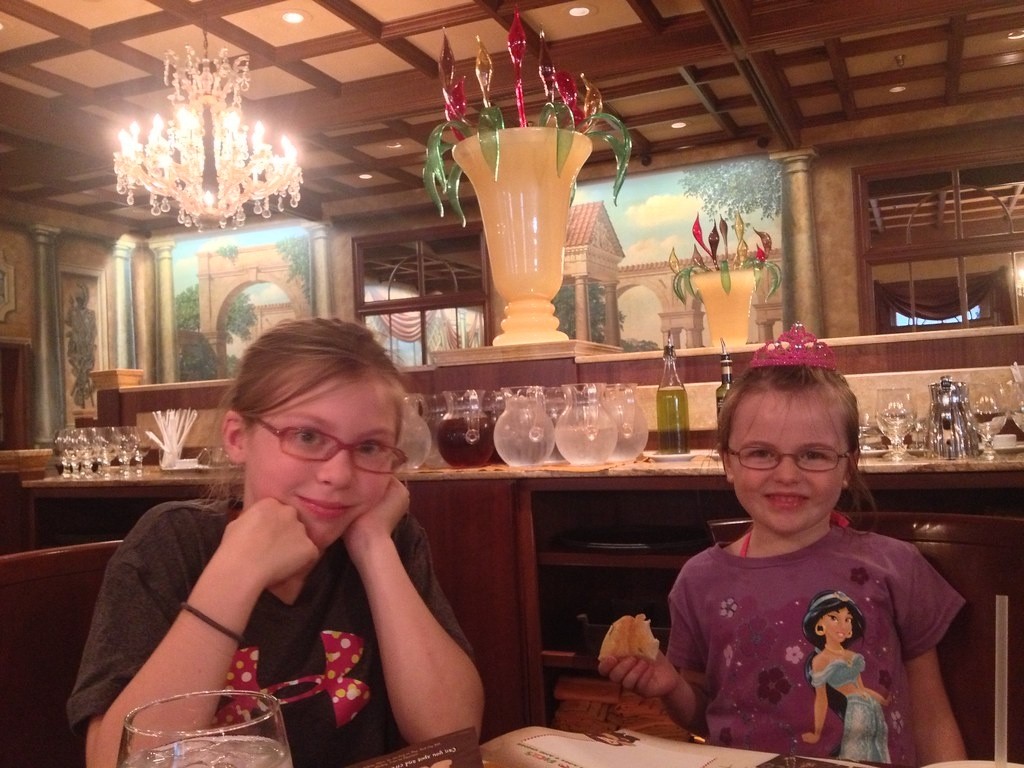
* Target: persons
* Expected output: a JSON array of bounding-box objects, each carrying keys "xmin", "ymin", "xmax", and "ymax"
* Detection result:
[
  {"xmin": 597, "ymin": 363, "xmax": 967, "ymax": 768},
  {"xmin": 68, "ymin": 317, "xmax": 482, "ymax": 768}
]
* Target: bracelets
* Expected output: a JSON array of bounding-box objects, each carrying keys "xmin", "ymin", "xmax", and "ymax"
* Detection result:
[{"xmin": 181, "ymin": 603, "xmax": 245, "ymax": 643}]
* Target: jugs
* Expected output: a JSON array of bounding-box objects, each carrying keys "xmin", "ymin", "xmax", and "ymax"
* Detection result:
[
  {"xmin": 926, "ymin": 375, "xmax": 980, "ymax": 460},
  {"xmin": 399, "ymin": 381, "xmax": 650, "ymax": 470}
]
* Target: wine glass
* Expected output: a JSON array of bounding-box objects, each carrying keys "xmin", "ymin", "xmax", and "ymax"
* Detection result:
[
  {"xmin": 966, "ymin": 380, "xmax": 1024, "ymax": 461},
  {"xmin": 53, "ymin": 425, "xmax": 154, "ymax": 480},
  {"xmin": 855, "ymin": 387, "xmax": 920, "ymax": 462}
]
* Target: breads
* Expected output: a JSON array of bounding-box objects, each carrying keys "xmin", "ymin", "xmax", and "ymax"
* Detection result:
[{"xmin": 598, "ymin": 613, "xmax": 659, "ymax": 661}]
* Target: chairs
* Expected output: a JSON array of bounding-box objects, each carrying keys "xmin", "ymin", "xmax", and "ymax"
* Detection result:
[
  {"xmin": 1, "ymin": 537, "xmax": 125, "ymax": 768},
  {"xmin": 704, "ymin": 513, "xmax": 1024, "ymax": 767},
  {"xmin": 112, "ymin": 6, "xmax": 302, "ymax": 227}
]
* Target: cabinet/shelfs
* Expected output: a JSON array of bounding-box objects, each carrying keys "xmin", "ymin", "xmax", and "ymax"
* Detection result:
[{"xmin": 23, "ymin": 460, "xmax": 1024, "ymax": 745}]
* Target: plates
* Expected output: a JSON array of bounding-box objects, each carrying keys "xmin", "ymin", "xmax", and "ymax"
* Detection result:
[
  {"xmin": 978, "ymin": 444, "xmax": 1024, "ymax": 454},
  {"xmin": 643, "ymin": 451, "xmax": 696, "ymax": 462},
  {"xmin": 860, "ymin": 449, "xmax": 892, "ymax": 457},
  {"xmin": 705, "ymin": 453, "xmax": 722, "ymax": 461},
  {"xmin": 196, "ymin": 465, "xmax": 240, "ymax": 470},
  {"xmin": 904, "ymin": 450, "xmax": 929, "ymax": 457}
]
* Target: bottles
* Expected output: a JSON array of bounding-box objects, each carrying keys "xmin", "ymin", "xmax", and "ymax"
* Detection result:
[
  {"xmin": 655, "ymin": 330, "xmax": 690, "ymax": 454},
  {"xmin": 715, "ymin": 337, "xmax": 735, "ymax": 422}
]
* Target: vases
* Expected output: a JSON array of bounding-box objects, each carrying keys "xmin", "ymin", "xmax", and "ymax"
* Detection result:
[
  {"xmin": 691, "ymin": 271, "xmax": 756, "ymax": 348},
  {"xmin": 450, "ymin": 129, "xmax": 593, "ymax": 346}
]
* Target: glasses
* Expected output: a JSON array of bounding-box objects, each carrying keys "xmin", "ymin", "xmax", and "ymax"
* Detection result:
[
  {"xmin": 724, "ymin": 445, "xmax": 851, "ymax": 472},
  {"xmin": 252, "ymin": 416, "xmax": 408, "ymax": 474}
]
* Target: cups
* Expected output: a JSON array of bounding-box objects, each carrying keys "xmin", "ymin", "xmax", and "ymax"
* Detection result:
[
  {"xmin": 116, "ymin": 690, "xmax": 294, "ymax": 768},
  {"xmin": 919, "ymin": 760, "xmax": 1024, "ymax": 768},
  {"xmin": 158, "ymin": 448, "xmax": 181, "ymax": 468},
  {"xmin": 211, "ymin": 447, "xmax": 228, "ymax": 466},
  {"xmin": 993, "ymin": 434, "xmax": 1016, "ymax": 448}
]
